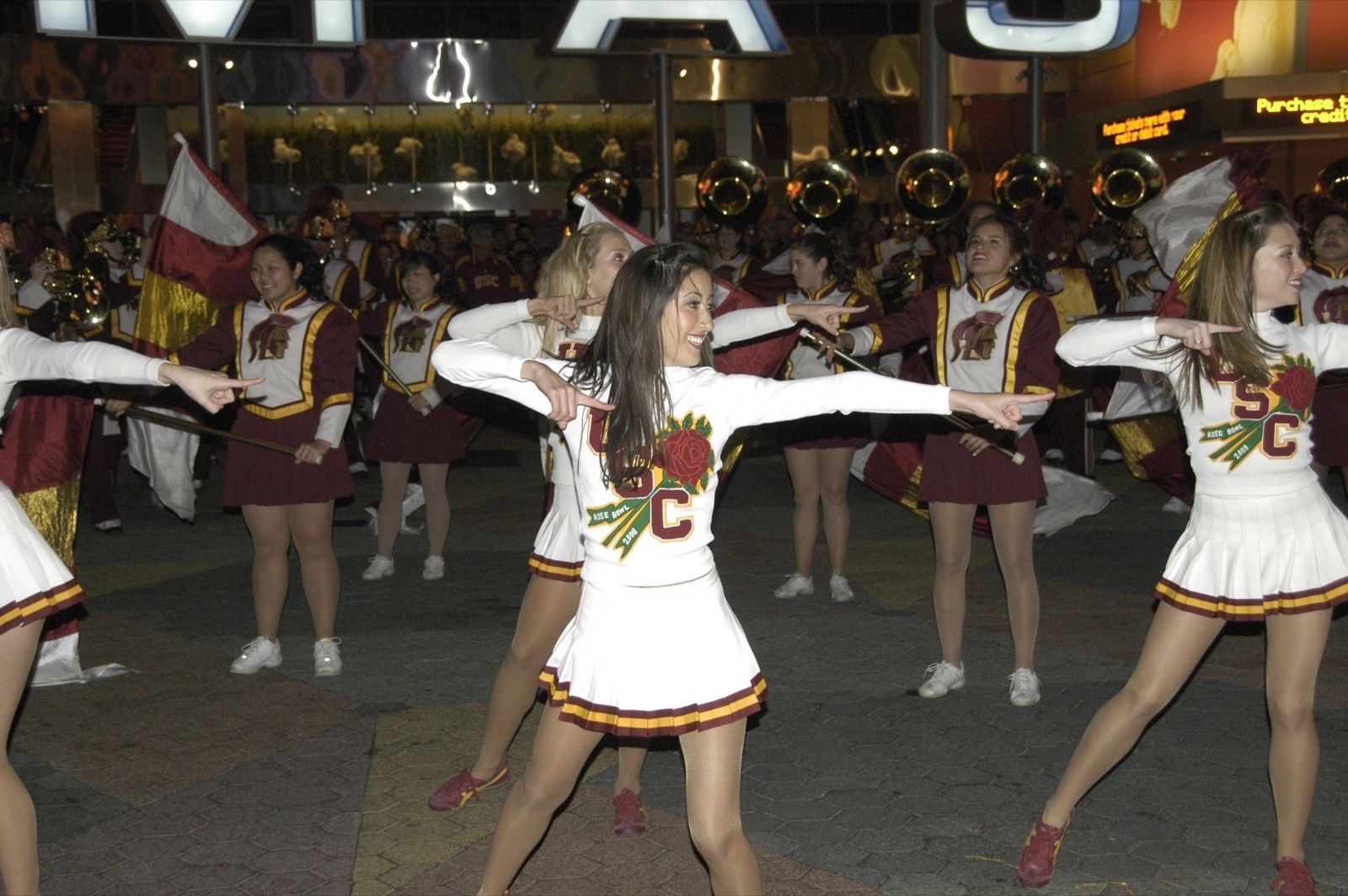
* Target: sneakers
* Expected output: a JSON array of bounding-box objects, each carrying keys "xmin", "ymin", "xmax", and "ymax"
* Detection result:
[
  {"xmin": 230, "ymin": 634, "xmax": 282, "ymax": 673},
  {"xmin": 917, "ymin": 660, "xmax": 965, "ymax": 698},
  {"xmin": 774, "ymin": 573, "xmax": 815, "ymax": 599},
  {"xmin": 421, "ymin": 555, "xmax": 446, "ymax": 580},
  {"xmin": 1007, "ymin": 668, "xmax": 1044, "ymax": 707},
  {"xmin": 428, "ymin": 759, "xmax": 510, "ymax": 811},
  {"xmin": 363, "ymin": 553, "xmax": 395, "ymax": 581},
  {"xmin": 612, "ymin": 788, "xmax": 648, "ymax": 837},
  {"xmin": 1270, "ymin": 857, "xmax": 1316, "ymax": 896},
  {"xmin": 829, "ymin": 567, "xmax": 854, "ymax": 603},
  {"xmin": 311, "ymin": 636, "xmax": 343, "ymax": 677},
  {"xmin": 1016, "ymin": 809, "xmax": 1073, "ymax": 888}
]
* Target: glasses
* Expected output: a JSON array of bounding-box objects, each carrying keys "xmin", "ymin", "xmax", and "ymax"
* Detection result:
[
  {"xmin": 332, "ymin": 216, "xmax": 347, "ymax": 222},
  {"xmin": 310, "ymin": 234, "xmax": 332, "ymax": 242},
  {"xmin": 901, "ymin": 223, "xmax": 915, "ymax": 228},
  {"xmin": 107, "ymin": 238, "xmax": 116, "ymax": 243},
  {"xmin": 1124, "ymin": 236, "xmax": 1144, "ymax": 240},
  {"xmin": 421, "ymin": 235, "xmax": 439, "ymax": 242}
]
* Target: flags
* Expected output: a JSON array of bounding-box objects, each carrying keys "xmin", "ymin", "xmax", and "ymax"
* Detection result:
[
  {"xmin": 1133, "ymin": 147, "xmax": 1270, "ymax": 317},
  {"xmin": 576, "ymin": 203, "xmax": 804, "ymax": 483},
  {"xmin": 126, "ymin": 148, "xmax": 265, "ymax": 521},
  {"xmin": 0, "ymin": 379, "xmax": 96, "ymax": 576}
]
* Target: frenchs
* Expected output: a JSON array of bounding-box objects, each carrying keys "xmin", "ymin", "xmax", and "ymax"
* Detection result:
[
  {"xmin": 566, "ymin": 170, "xmax": 641, "ymax": 231},
  {"xmin": 895, "ymin": 148, "xmax": 971, "ymax": 223},
  {"xmin": 785, "ymin": 160, "xmax": 858, "ymax": 229},
  {"xmin": 1087, "ymin": 148, "xmax": 1167, "ymax": 222},
  {"xmin": 992, "ymin": 153, "xmax": 1065, "ymax": 217},
  {"xmin": 695, "ymin": 157, "xmax": 768, "ymax": 232}
]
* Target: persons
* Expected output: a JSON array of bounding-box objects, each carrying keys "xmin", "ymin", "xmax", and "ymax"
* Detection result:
[
  {"xmin": 0, "ymin": 240, "xmax": 265, "ymax": 896},
  {"xmin": 427, "ymin": 222, "xmax": 870, "ymax": 836},
  {"xmin": 771, "ymin": 235, "xmax": 881, "ymax": 602},
  {"xmin": 812, "ymin": 200, "xmax": 1058, "ymax": 706},
  {"xmin": 1018, "ymin": 202, "xmax": 1348, "ymax": 896},
  {"xmin": 430, "ymin": 242, "xmax": 1056, "ymax": 896},
  {"xmin": 1290, "ymin": 209, "xmax": 1348, "ymax": 520},
  {"xmin": 359, "ymin": 251, "xmax": 466, "ymax": 581},
  {"xmin": 300, "ymin": 199, "xmax": 556, "ymax": 478},
  {"xmin": 105, "ymin": 233, "xmax": 358, "ymax": 677},
  {"xmin": 1045, "ymin": 217, "xmax": 1171, "ymax": 463},
  {"xmin": 0, "ymin": 211, "xmax": 151, "ymax": 538},
  {"xmin": 703, "ymin": 222, "xmax": 765, "ymax": 286}
]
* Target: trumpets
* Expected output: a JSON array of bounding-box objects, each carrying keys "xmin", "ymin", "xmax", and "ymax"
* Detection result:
[{"xmin": 41, "ymin": 267, "xmax": 112, "ymax": 331}]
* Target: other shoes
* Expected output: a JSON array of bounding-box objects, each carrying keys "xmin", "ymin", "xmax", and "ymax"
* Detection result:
[
  {"xmin": 95, "ymin": 519, "xmax": 122, "ymax": 535},
  {"xmin": 193, "ymin": 480, "xmax": 202, "ymax": 488},
  {"xmin": 349, "ymin": 462, "xmax": 368, "ymax": 477},
  {"xmin": 1044, "ymin": 450, "xmax": 1064, "ymax": 465},
  {"xmin": 1160, "ymin": 495, "xmax": 1191, "ymax": 514},
  {"xmin": 1096, "ymin": 449, "xmax": 1124, "ymax": 463}
]
